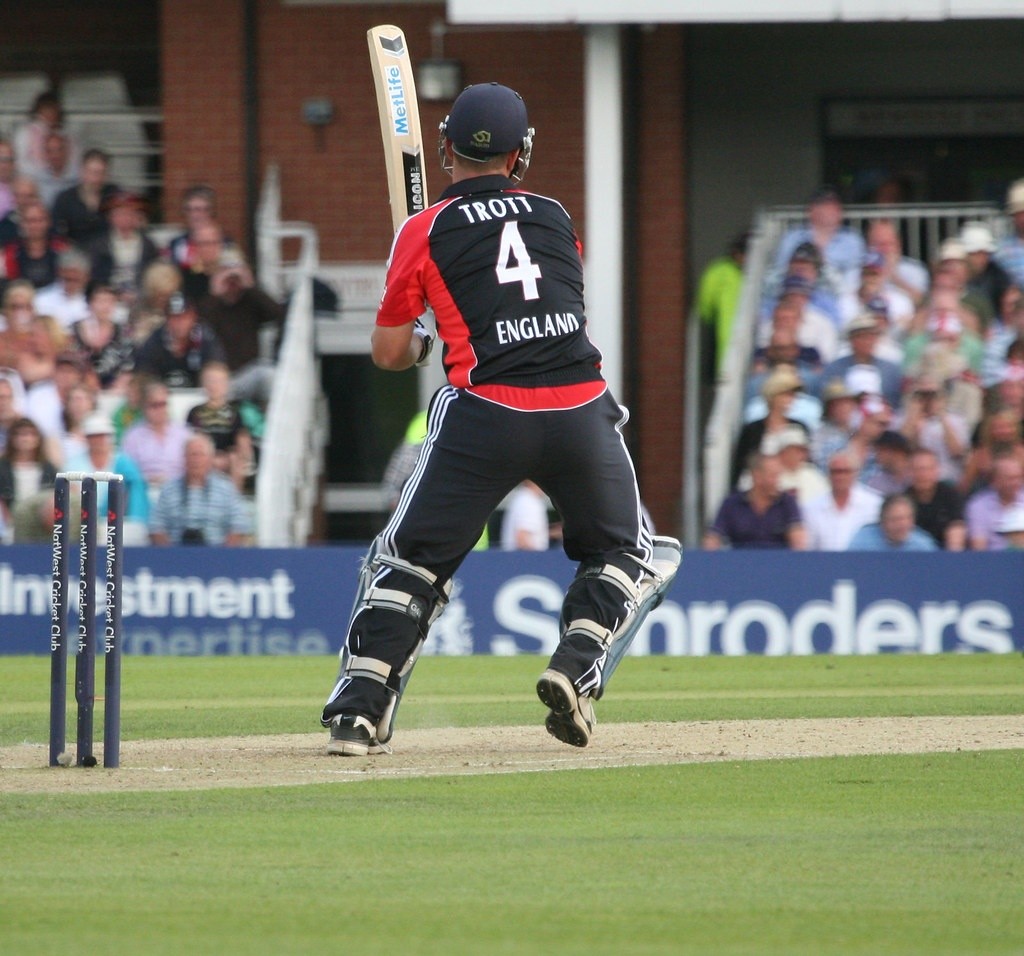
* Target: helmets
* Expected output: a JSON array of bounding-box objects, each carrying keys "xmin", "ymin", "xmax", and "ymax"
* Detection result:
[{"xmin": 439, "ymin": 81, "xmax": 534, "ymax": 164}]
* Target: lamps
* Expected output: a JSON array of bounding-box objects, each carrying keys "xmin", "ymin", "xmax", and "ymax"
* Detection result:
[{"xmin": 416, "ymin": 57, "xmax": 462, "ymax": 101}]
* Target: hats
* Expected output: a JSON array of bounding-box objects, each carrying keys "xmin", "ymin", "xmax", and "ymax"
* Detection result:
[{"xmin": 755, "ymin": 174, "xmax": 1022, "ymax": 419}]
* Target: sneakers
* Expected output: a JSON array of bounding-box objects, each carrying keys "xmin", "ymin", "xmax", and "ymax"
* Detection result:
[
  {"xmin": 324, "ymin": 710, "xmax": 387, "ymax": 757},
  {"xmin": 536, "ymin": 669, "xmax": 592, "ymax": 748}
]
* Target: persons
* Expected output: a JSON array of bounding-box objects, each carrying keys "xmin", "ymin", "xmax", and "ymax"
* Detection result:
[
  {"xmin": 693, "ymin": 175, "xmax": 1024, "ymax": 548},
  {"xmin": 1, "ymin": 92, "xmax": 335, "ymax": 547},
  {"xmin": 321, "ymin": 83, "xmax": 650, "ymax": 757},
  {"xmin": 384, "ymin": 408, "xmax": 549, "ymax": 552}
]
